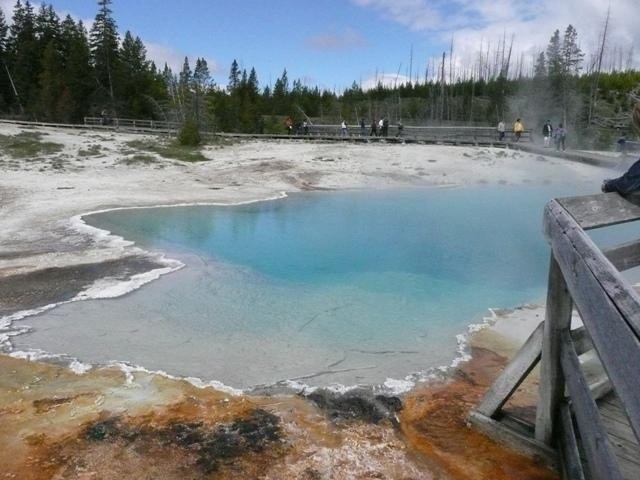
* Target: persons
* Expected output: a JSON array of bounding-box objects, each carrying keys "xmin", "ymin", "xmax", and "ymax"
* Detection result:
[
  {"xmin": 555, "ymin": 124, "xmax": 566, "ymax": 152},
  {"xmin": 396, "ymin": 119, "xmax": 405, "ymax": 139},
  {"xmin": 497, "ymin": 120, "xmax": 506, "ymax": 141},
  {"xmin": 541, "ymin": 119, "xmax": 553, "ymax": 147},
  {"xmin": 360, "ymin": 117, "xmax": 389, "ymax": 137},
  {"xmin": 514, "ymin": 118, "xmax": 524, "ymax": 143},
  {"xmin": 285, "ymin": 117, "xmax": 293, "ymax": 135},
  {"xmin": 340, "ymin": 120, "xmax": 348, "ymax": 135},
  {"xmin": 304, "ymin": 118, "xmax": 308, "ymax": 132}
]
